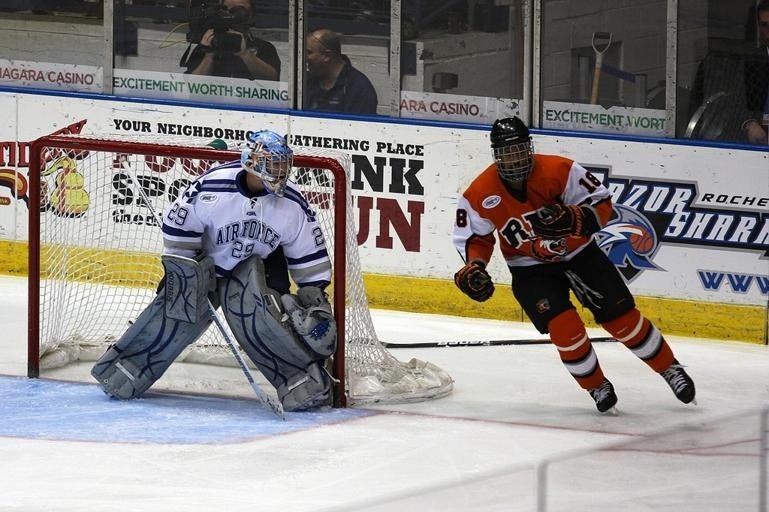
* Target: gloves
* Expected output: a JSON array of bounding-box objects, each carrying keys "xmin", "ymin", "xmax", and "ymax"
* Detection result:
[
  {"xmin": 527, "ymin": 193, "xmax": 590, "ymax": 240},
  {"xmin": 454, "ymin": 257, "xmax": 495, "ymax": 302}
]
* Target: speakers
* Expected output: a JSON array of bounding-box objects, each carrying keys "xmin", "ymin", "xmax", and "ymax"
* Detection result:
[
  {"xmin": 388, "ymin": 42, "xmax": 416, "ymax": 75},
  {"xmin": 113, "ymin": 21, "xmax": 136, "ymax": 56}
]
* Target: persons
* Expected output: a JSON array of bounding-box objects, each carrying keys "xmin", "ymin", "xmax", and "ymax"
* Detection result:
[
  {"xmin": 739, "ymin": 4, "xmax": 768, "ymax": 146},
  {"xmin": 450, "ymin": 116, "xmax": 697, "ymax": 413},
  {"xmin": 85, "ymin": 131, "xmax": 338, "ymax": 412},
  {"xmin": 305, "ymin": 29, "xmax": 379, "ymax": 116},
  {"xmin": 183, "ymin": 1, "xmax": 281, "ymax": 85}
]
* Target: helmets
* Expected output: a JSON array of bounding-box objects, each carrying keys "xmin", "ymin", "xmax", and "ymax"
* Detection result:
[
  {"xmin": 239, "ymin": 130, "xmax": 295, "ymax": 198},
  {"xmin": 489, "ymin": 115, "xmax": 536, "ymax": 183}
]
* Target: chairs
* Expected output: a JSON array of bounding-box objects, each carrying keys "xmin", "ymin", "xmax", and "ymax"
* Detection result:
[{"xmin": 645, "ymin": 49, "xmax": 745, "ymax": 141}]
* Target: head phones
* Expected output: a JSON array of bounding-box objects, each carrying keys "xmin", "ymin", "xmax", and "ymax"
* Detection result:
[{"xmin": 221, "ymin": 0, "xmax": 256, "ymax": 27}]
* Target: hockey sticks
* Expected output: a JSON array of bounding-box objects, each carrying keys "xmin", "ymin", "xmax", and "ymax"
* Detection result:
[
  {"xmin": 347, "ymin": 336, "xmax": 619, "ymax": 352},
  {"xmin": 120, "ymin": 155, "xmax": 286, "ymax": 422}
]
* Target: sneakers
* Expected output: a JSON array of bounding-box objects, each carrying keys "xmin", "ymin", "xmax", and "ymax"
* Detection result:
[
  {"xmin": 584, "ymin": 378, "xmax": 619, "ymax": 413},
  {"xmin": 658, "ymin": 358, "xmax": 695, "ymax": 403}
]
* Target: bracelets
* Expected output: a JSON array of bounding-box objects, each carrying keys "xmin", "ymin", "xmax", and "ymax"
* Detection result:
[{"xmin": 741, "ymin": 119, "xmax": 758, "ymax": 132}]
